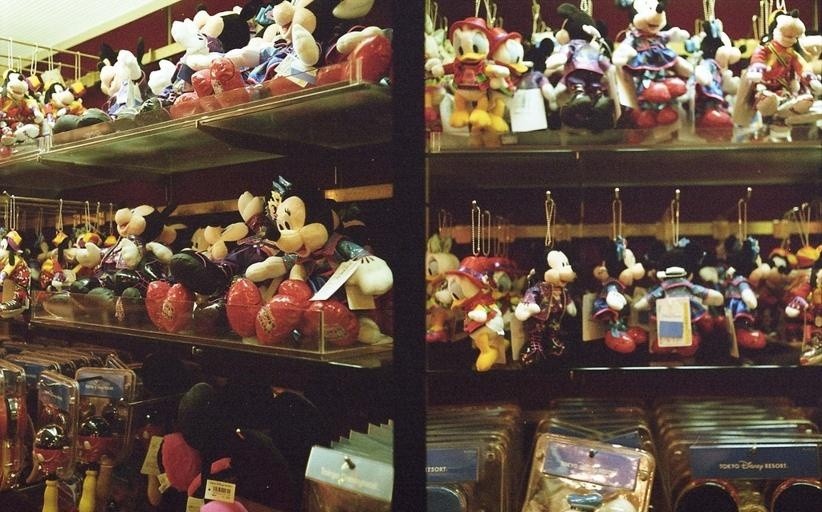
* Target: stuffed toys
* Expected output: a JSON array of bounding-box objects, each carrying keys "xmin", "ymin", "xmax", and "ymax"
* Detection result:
[
  {"xmin": 425, "ymin": 0, "xmax": 822, "ymax": 512},
  {"xmin": 0, "ymin": 0, "xmax": 400, "ymax": 512}
]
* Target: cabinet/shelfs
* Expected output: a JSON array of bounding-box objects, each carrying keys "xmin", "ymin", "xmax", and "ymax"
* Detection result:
[
  {"xmin": 0, "ymin": 55, "xmax": 408, "ymax": 370},
  {"xmin": 407, "ymin": 115, "xmax": 821, "ymax": 412}
]
